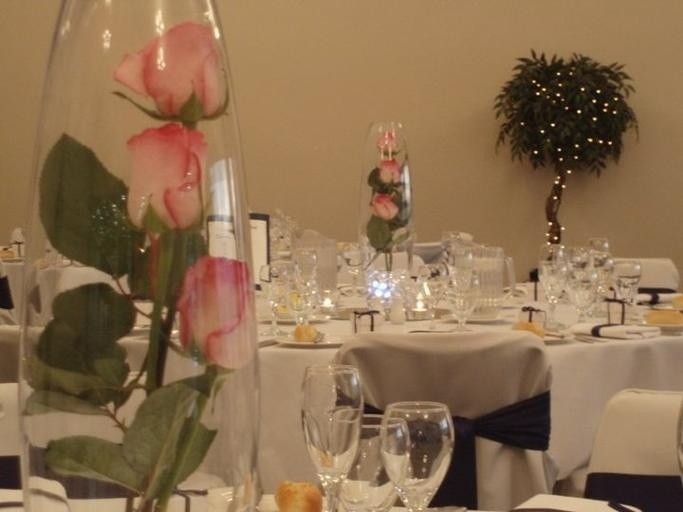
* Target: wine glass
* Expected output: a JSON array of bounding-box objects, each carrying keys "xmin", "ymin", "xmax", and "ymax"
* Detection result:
[
  {"xmin": 538, "ymin": 261, "xmax": 568, "ymax": 331},
  {"xmin": 569, "ymin": 247, "xmax": 590, "ymax": 261},
  {"xmin": 379, "ymin": 401, "xmax": 455, "ymax": 510},
  {"xmin": 451, "ymin": 267, "xmax": 479, "ymax": 331},
  {"xmin": 613, "ymin": 260, "xmax": 642, "ymax": 305},
  {"xmin": 258, "ymin": 264, "xmax": 293, "ymax": 338},
  {"xmin": 300, "ymin": 364, "xmax": 364, "ymax": 511},
  {"xmin": 538, "ymin": 244, "xmax": 566, "ymax": 264},
  {"xmin": 588, "ymin": 238, "xmax": 614, "ymax": 294},
  {"xmin": 335, "ymin": 415, "xmax": 410, "ymax": 510},
  {"xmin": 569, "ymin": 259, "xmax": 597, "ymax": 324},
  {"xmin": 418, "ymin": 265, "xmax": 449, "ymax": 329},
  {"xmin": 284, "ymin": 287, "xmax": 323, "ymax": 342}
]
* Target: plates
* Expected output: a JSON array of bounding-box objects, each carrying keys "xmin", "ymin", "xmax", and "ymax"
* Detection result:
[
  {"xmin": 642, "ymin": 310, "xmax": 682, "ymax": 328},
  {"xmin": 514, "ymin": 322, "xmax": 576, "ymax": 341},
  {"xmin": 653, "ymin": 302, "xmax": 683, "ymax": 310},
  {"xmin": 278, "ymin": 336, "xmax": 342, "ymax": 345}
]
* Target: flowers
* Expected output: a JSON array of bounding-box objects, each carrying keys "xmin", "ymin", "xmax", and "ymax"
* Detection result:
[{"xmin": 365, "ymin": 127, "xmax": 411, "ymax": 276}]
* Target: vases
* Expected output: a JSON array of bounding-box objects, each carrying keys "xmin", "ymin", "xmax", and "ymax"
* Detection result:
[{"xmin": 356, "ymin": 121, "xmax": 414, "ymax": 280}]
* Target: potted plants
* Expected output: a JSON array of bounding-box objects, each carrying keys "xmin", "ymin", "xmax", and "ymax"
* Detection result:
[{"xmin": 489, "ymin": 48, "xmax": 641, "ymax": 283}]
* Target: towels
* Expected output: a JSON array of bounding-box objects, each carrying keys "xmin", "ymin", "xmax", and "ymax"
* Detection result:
[
  {"xmin": 508, "ymin": 493, "xmax": 642, "ymax": 512},
  {"xmin": 571, "ymin": 323, "xmax": 661, "ymax": 339},
  {"xmin": 637, "ymin": 293, "xmax": 682, "ymax": 304}
]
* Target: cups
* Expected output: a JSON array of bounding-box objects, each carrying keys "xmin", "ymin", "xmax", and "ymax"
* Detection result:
[{"xmin": 457, "ymin": 244, "xmax": 515, "ymax": 318}]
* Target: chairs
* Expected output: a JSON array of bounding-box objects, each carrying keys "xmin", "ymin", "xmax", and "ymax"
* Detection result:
[
  {"xmin": 439, "ymin": 255, "xmax": 515, "ymax": 295},
  {"xmin": 602, "ymin": 256, "xmax": 680, "ymax": 299},
  {"xmin": 331, "ymin": 323, "xmax": 553, "ymax": 511},
  {"xmin": 333, "ymin": 252, "xmax": 429, "ymax": 287},
  {"xmin": 36, "ymin": 266, "xmax": 127, "ymax": 326},
  {"xmin": 580, "ymin": 387, "xmax": 683, "ymax": 511}
]
